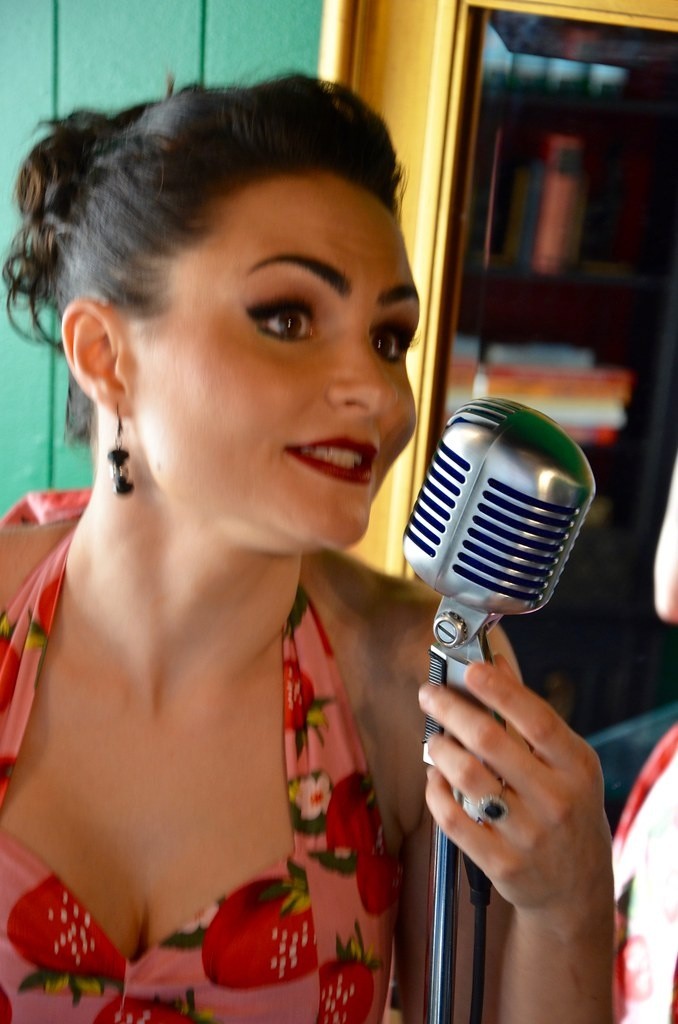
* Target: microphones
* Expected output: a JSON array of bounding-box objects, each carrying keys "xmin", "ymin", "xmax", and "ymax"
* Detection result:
[{"xmin": 402, "ymin": 394, "xmax": 595, "ymax": 908}]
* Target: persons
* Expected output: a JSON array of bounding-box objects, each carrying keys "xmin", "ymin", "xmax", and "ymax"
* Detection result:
[{"xmin": 0, "ymin": 70, "xmax": 678, "ymax": 1024}]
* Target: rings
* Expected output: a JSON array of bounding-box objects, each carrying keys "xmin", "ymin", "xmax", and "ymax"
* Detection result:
[{"xmin": 465, "ymin": 778, "xmax": 508, "ymax": 825}]
